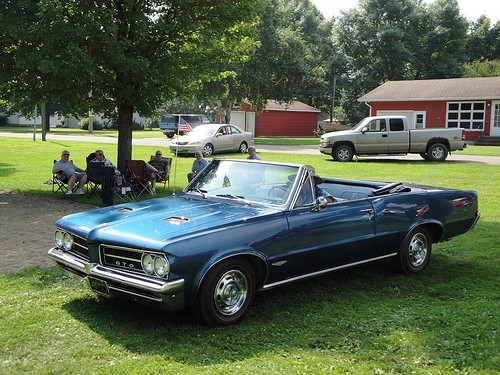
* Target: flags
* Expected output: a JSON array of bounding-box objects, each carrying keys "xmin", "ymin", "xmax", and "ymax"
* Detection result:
[{"xmin": 179, "ymin": 117, "xmax": 193, "ymax": 133}]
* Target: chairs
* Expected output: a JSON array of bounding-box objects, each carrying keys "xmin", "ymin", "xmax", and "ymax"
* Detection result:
[{"xmin": 53, "ymin": 153, "xmax": 172, "ymax": 203}]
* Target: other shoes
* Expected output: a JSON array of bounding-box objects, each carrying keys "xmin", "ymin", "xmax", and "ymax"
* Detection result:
[
  {"xmin": 76, "ymin": 190, "xmax": 84, "ymax": 194},
  {"xmin": 153, "ymin": 191, "xmax": 156, "ymax": 194}
]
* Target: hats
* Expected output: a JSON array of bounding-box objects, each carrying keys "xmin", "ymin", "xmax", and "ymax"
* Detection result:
[
  {"xmin": 288, "ymin": 165, "xmax": 323, "ymax": 185},
  {"xmin": 62, "ymin": 151, "xmax": 70, "ymax": 155}
]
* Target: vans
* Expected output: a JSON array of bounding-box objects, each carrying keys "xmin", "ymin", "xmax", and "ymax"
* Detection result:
[{"xmin": 159, "ymin": 113, "xmax": 212, "ymax": 138}]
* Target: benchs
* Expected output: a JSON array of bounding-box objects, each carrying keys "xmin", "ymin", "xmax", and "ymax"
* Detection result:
[{"xmin": 319, "ymin": 183, "xmax": 374, "ymax": 201}]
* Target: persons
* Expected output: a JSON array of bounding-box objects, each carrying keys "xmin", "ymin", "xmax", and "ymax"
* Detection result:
[
  {"xmin": 52, "ymin": 150, "xmax": 88, "ymax": 195},
  {"xmin": 282, "ymin": 165, "xmax": 337, "ymax": 203},
  {"xmin": 90, "ymin": 149, "xmax": 121, "ymax": 193},
  {"xmin": 247, "ymin": 146, "xmax": 265, "ymax": 182},
  {"xmin": 187, "ymin": 151, "xmax": 208, "ymax": 183},
  {"xmin": 146, "ymin": 150, "xmax": 170, "ymax": 194}
]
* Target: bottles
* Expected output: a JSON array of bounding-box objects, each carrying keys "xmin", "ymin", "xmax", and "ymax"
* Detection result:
[{"xmin": 122, "ymin": 186, "xmax": 126, "ymax": 194}]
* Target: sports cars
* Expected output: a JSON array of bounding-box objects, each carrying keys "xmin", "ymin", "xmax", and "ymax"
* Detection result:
[{"xmin": 46, "ymin": 159, "xmax": 482, "ymax": 328}]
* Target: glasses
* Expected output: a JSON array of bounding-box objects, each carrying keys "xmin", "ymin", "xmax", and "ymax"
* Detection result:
[
  {"xmin": 98, "ymin": 154, "xmax": 103, "ymax": 155},
  {"xmin": 156, "ymin": 154, "xmax": 161, "ymax": 155}
]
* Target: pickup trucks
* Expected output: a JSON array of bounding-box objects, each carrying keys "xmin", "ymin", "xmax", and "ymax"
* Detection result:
[{"xmin": 318, "ymin": 115, "xmax": 469, "ymax": 164}]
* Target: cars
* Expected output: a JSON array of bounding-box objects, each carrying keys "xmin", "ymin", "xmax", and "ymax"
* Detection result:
[{"xmin": 169, "ymin": 123, "xmax": 254, "ymax": 157}]
